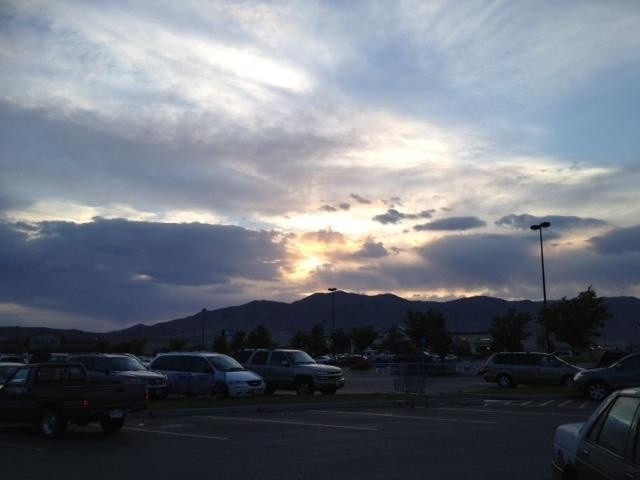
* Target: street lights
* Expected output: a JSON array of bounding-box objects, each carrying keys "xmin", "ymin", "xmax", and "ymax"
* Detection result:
[
  {"xmin": 530, "ymin": 222, "xmax": 552, "ymax": 350},
  {"xmin": 327, "ymin": 286, "xmax": 338, "ymax": 359}
]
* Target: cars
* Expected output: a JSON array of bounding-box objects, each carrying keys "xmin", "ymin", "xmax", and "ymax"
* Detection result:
[
  {"xmin": 550, "ymin": 387, "xmax": 640, "ymax": 480},
  {"xmin": 572, "ymin": 350, "xmax": 639, "ymax": 400},
  {"xmin": 316, "ymin": 350, "xmax": 456, "ymax": 362}
]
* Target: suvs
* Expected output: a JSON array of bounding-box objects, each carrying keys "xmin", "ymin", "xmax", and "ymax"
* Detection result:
[
  {"xmin": 64, "ymin": 353, "xmax": 172, "ymax": 403},
  {"xmin": 479, "ymin": 351, "xmax": 587, "ymax": 389},
  {"xmin": 230, "ymin": 348, "xmax": 345, "ymax": 398},
  {"xmin": 146, "ymin": 352, "xmax": 266, "ymax": 402}
]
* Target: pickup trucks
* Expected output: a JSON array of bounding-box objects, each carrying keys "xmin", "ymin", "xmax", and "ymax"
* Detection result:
[{"xmin": 0, "ymin": 362, "xmax": 148, "ymax": 440}]
